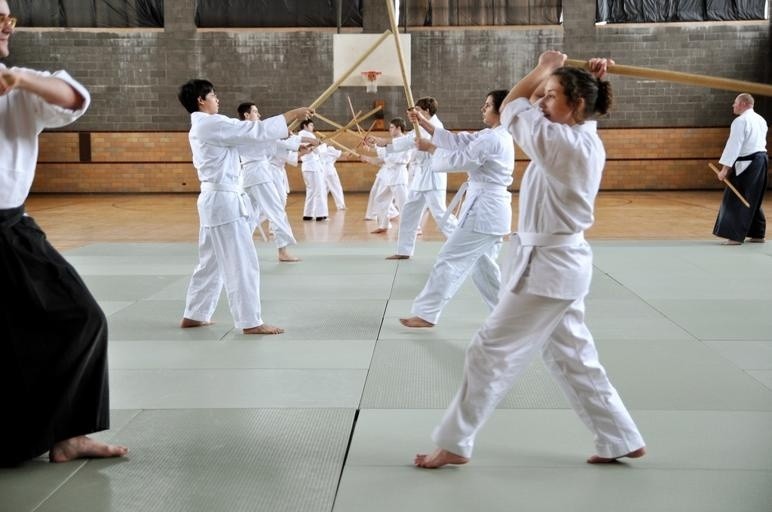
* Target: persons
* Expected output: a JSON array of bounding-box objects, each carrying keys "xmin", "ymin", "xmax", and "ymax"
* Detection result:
[
  {"xmin": 235, "ymin": 100, "xmax": 324, "ymax": 264},
  {"xmin": 362, "ymin": 95, "xmax": 459, "ymax": 260},
  {"xmin": 0, "ymin": 0, "xmax": 133, "ymax": 473},
  {"xmin": 410, "ymin": 47, "xmax": 648, "ymax": 470},
  {"xmin": 397, "ymin": 88, "xmax": 517, "ymax": 329},
  {"xmin": 318, "ymin": 135, "xmax": 357, "ymax": 213},
  {"xmin": 296, "ymin": 119, "xmax": 329, "ymax": 221},
  {"xmin": 357, "ymin": 141, "xmax": 399, "ymax": 222},
  {"xmin": 362, "ymin": 116, "xmax": 422, "ymax": 236},
  {"xmin": 711, "ymin": 92, "xmax": 768, "ymax": 247},
  {"xmin": 175, "ymin": 77, "xmax": 317, "ymax": 336},
  {"xmin": 266, "ymin": 138, "xmax": 312, "ymax": 241}
]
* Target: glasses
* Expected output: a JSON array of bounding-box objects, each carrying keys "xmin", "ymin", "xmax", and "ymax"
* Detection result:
[{"xmin": 0, "ymin": 16, "xmax": 17, "ymax": 28}]
